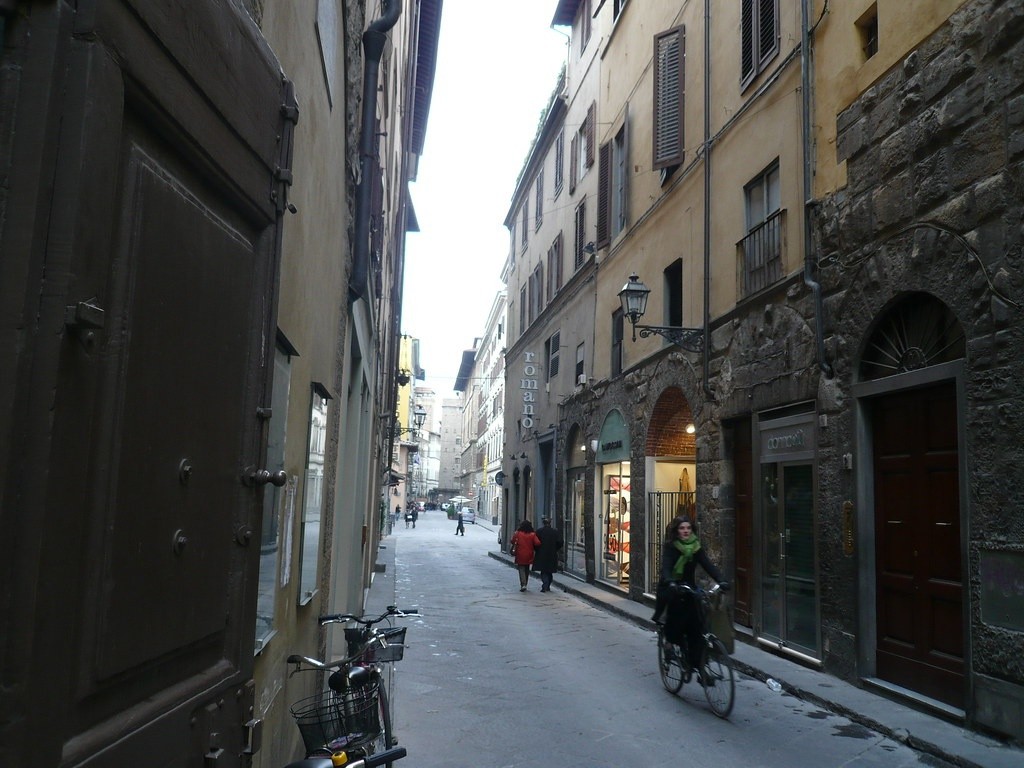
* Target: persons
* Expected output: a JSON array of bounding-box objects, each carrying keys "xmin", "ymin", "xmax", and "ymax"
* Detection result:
[
  {"xmin": 511, "ymin": 520, "xmax": 542, "ymax": 592},
  {"xmin": 652, "ymin": 514, "xmax": 731, "ymax": 686},
  {"xmin": 395, "ymin": 504, "xmax": 418, "ymax": 528},
  {"xmin": 532, "ymin": 518, "xmax": 563, "ymax": 592},
  {"xmin": 455, "ymin": 512, "xmax": 464, "ymax": 536}
]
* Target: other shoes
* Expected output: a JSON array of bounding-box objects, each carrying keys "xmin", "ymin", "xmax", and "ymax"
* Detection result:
[{"xmin": 520, "ymin": 585, "xmax": 527, "ymax": 592}]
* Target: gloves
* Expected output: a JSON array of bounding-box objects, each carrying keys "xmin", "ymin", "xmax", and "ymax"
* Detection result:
[{"xmin": 718, "ymin": 581, "xmax": 731, "ymax": 594}]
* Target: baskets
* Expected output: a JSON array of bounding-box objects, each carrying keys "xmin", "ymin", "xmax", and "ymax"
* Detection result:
[
  {"xmin": 343, "ymin": 627, "xmax": 407, "ymax": 662},
  {"xmin": 289, "ymin": 681, "xmax": 380, "ymax": 754}
]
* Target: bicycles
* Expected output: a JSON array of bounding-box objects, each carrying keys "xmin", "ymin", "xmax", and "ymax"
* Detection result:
[
  {"xmin": 281, "ymin": 605, "xmax": 425, "ymax": 768},
  {"xmin": 656, "ymin": 582, "xmax": 736, "ymax": 719}
]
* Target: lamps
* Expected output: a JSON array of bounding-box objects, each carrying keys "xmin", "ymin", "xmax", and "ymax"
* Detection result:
[{"xmin": 617, "ymin": 272, "xmax": 704, "ymax": 353}]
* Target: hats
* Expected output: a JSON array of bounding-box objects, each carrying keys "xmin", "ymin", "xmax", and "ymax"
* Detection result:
[{"xmin": 458, "ymin": 512, "xmax": 462, "ymax": 515}]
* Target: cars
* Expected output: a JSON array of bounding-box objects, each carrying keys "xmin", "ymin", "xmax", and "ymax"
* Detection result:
[
  {"xmin": 416, "ymin": 502, "xmax": 425, "ymax": 511},
  {"xmin": 461, "ymin": 507, "xmax": 476, "ymax": 524}
]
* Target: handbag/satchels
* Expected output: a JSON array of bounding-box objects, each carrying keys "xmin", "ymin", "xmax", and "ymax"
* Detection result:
[
  {"xmin": 461, "ymin": 527, "xmax": 466, "ymax": 532},
  {"xmin": 663, "ymin": 590, "xmax": 703, "ymax": 644},
  {"xmin": 707, "ymin": 595, "xmax": 735, "ymax": 655},
  {"xmin": 406, "ymin": 515, "xmax": 412, "ymax": 521},
  {"xmin": 510, "ymin": 531, "xmax": 517, "ymax": 555}
]
